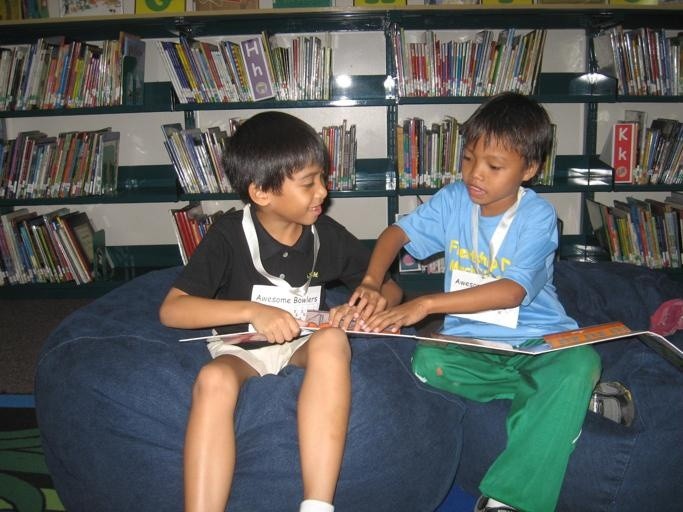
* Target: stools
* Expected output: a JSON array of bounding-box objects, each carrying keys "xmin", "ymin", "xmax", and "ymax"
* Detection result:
[
  {"xmin": 472, "ymin": 261, "xmax": 681, "ymax": 511},
  {"xmin": 32, "ymin": 263, "xmax": 467, "ymax": 512}
]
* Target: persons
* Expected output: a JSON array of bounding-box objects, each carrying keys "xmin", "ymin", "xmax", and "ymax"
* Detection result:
[
  {"xmin": 348, "ymin": 91, "xmax": 635, "ymax": 511},
  {"xmin": 158, "ymin": 112, "xmax": 405, "ymax": 511}
]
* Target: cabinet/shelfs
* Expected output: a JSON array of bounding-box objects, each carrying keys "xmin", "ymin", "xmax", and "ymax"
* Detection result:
[{"xmin": 1, "ymin": 1, "xmax": 683, "ymax": 297}]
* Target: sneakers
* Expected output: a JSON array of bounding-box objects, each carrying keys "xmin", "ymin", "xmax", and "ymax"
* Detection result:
[
  {"xmin": 585, "ymin": 381, "xmax": 636, "ymax": 428},
  {"xmin": 474, "ymin": 493, "xmax": 515, "ymax": 511}
]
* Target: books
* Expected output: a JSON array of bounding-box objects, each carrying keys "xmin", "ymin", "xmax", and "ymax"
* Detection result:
[
  {"xmin": 0, "ymin": 31, "xmax": 145, "ymax": 108},
  {"xmin": 593, "ymin": 22, "xmax": 683, "ymax": 96},
  {"xmin": 0, "ymin": 208, "xmax": 115, "ymax": 285},
  {"xmin": 396, "ymin": 115, "xmax": 557, "ymax": 189},
  {"xmin": 600, "ymin": 110, "xmax": 683, "ymax": 186},
  {"xmin": 422, "ymin": 253, "xmax": 446, "ymax": 274},
  {"xmin": 586, "ymin": 193, "xmax": 683, "ymax": 269},
  {"xmin": 161, "ymin": 118, "xmax": 357, "ymax": 193},
  {"xmin": 168, "ymin": 203, "xmax": 235, "ymax": 266},
  {"xmin": 154, "ymin": 22, "xmax": 332, "ymax": 105},
  {"xmin": 0, "ymin": 127, "xmax": 120, "ymax": 199},
  {"xmin": 389, "ymin": 22, "xmax": 548, "ymax": 94}
]
